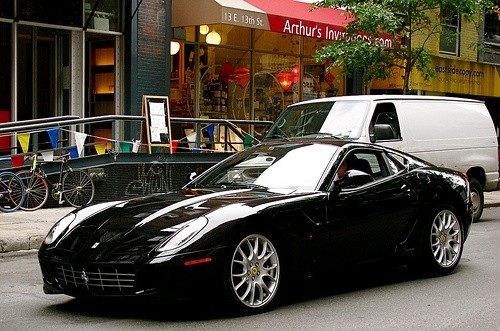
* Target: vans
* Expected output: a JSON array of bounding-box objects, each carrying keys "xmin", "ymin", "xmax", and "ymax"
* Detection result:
[{"xmin": 232, "ymin": 93, "xmax": 499, "ymax": 222}]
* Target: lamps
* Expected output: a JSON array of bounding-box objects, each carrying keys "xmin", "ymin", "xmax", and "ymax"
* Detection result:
[
  {"xmin": 199, "ymin": 24, "xmax": 210, "ymax": 35},
  {"xmin": 279, "ymin": 64, "xmax": 300, "ymax": 93},
  {"xmin": 205, "ymin": 24, "xmax": 221, "ymax": 45},
  {"xmin": 170, "ymin": 41, "xmax": 180, "ymax": 55}
]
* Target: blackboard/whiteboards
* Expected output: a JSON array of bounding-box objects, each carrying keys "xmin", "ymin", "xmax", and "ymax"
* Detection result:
[{"xmin": 144, "ymin": 96, "xmax": 174, "ymax": 147}]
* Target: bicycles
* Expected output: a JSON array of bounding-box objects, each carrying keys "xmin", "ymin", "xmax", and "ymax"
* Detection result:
[
  {"xmin": 8, "ymin": 150, "xmax": 95, "ymax": 211},
  {"xmin": 0, "ymin": 171, "xmax": 25, "ymax": 213}
]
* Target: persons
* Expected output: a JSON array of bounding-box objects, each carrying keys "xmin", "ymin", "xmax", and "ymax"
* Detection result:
[{"xmin": 326, "ymin": 156, "xmax": 350, "ymax": 191}]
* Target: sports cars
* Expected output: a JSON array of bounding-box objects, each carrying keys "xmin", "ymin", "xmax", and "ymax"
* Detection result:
[{"xmin": 37, "ymin": 132, "xmax": 474, "ymax": 318}]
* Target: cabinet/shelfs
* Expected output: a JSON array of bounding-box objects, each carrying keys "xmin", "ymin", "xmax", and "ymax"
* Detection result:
[
  {"xmin": 165, "ymin": 41, "xmax": 210, "ymax": 100},
  {"xmin": 303, "ymin": 65, "xmax": 326, "ymax": 83}
]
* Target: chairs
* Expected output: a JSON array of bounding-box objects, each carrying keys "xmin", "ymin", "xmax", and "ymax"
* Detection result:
[{"xmin": 350, "ymin": 155, "xmax": 376, "ymax": 188}]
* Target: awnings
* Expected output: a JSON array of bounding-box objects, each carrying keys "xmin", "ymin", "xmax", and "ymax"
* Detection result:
[
  {"xmin": 172, "ymin": 0, "xmax": 271, "ymax": 30},
  {"xmin": 244, "ymin": 0, "xmax": 394, "ymax": 48}
]
